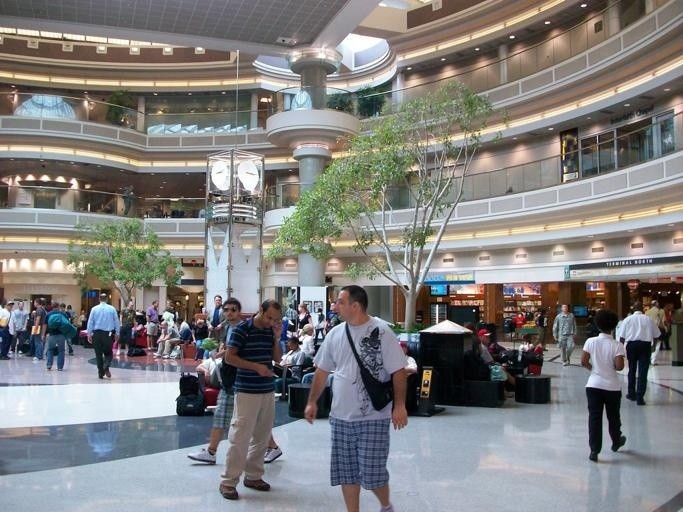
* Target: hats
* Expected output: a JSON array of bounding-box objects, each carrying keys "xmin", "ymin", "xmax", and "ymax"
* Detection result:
[
  {"xmin": 478, "ymin": 329, "xmax": 492, "ymax": 337},
  {"xmin": 7, "ymin": 301, "xmax": 14, "ymax": 306}
]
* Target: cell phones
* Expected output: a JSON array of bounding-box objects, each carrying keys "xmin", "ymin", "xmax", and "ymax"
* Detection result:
[{"xmin": 274, "ymin": 322, "xmax": 280, "ymax": 328}]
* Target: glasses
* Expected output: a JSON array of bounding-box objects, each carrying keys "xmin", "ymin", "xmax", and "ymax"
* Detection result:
[{"xmin": 223, "ymin": 307, "xmax": 237, "ymax": 312}]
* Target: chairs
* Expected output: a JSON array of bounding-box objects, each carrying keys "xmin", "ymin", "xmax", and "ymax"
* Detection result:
[{"xmin": 275, "ymin": 357, "xmax": 311, "ymax": 401}]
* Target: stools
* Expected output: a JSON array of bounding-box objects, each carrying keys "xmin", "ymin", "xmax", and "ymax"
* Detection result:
[
  {"xmin": 288, "ymin": 383, "xmax": 331, "ymax": 419},
  {"xmin": 515, "ymin": 374, "xmax": 551, "ymax": 404}
]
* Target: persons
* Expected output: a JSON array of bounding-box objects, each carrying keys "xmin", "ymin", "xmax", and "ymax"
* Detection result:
[
  {"xmin": 505, "ymin": 187, "xmax": 513, "ymax": 193},
  {"xmin": 219, "ymin": 298, "xmax": 283, "ymax": 500},
  {"xmin": 186, "ymin": 298, "xmax": 283, "ymax": 464},
  {"xmin": 618, "ymin": 298, "xmax": 673, "ymax": 406},
  {"xmin": 0, "ymin": 293, "xmax": 228, "ymax": 396},
  {"xmin": 272, "ymin": 302, "xmax": 342, "ymax": 399},
  {"xmin": 162, "ymin": 211, "xmax": 171, "ymax": 218},
  {"xmin": 399, "ymin": 342, "xmax": 418, "ymax": 382},
  {"xmin": 580, "ymin": 309, "xmax": 626, "ymax": 460},
  {"xmin": 143, "ymin": 211, "xmax": 151, "ymax": 217},
  {"xmin": 552, "ymin": 303, "xmax": 577, "ymax": 366},
  {"xmin": 122, "ymin": 185, "xmax": 135, "ymax": 215},
  {"xmin": 303, "ymin": 285, "xmax": 411, "ymax": 512},
  {"xmin": 470, "ymin": 306, "xmax": 550, "ymax": 401}
]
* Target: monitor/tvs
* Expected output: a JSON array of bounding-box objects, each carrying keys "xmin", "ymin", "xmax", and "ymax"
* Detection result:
[{"xmin": 430, "ymin": 285, "xmax": 449, "ymax": 296}]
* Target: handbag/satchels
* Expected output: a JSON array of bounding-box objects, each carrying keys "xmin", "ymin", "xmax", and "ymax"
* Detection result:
[
  {"xmin": 181, "ymin": 375, "xmax": 200, "ymax": 395},
  {"xmin": 522, "ymin": 352, "xmax": 543, "ymax": 366},
  {"xmin": 203, "ymin": 337, "xmax": 217, "ymax": 349},
  {"xmin": 489, "ymin": 365, "xmax": 509, "ymax": 382},
  {"xmin": 0, "ymin": 328, "xmax": 9, "ymax": 337},
  {"xmin": 127, "ymin": 347, "xmax": 146, "ymax": 356},
  {"xmin": 658, "ymin": 327, "xmax": 666, "ymax": 341},
  {"xmin": 221, "ymin": 364, "xmax": 237, "ymax": 389},
  {"xmin": 360, "ymin": 368, "xmax": 394, "ymax": 412},
  {"xmin": 170, "ymin": 348, "xmax": 181, "ymax": 359},
  {"xmin": 176, "ymin": 395, "xmax": 204, "ymax": 416},
  {"xmin": 48, "ymin": 313, "xmax": 76, "ymax": 338}
]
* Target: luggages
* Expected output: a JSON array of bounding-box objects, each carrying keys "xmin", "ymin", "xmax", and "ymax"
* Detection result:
[{"xmin": 526, "ymin": 339, "xmax": 541, "ymax": 375}]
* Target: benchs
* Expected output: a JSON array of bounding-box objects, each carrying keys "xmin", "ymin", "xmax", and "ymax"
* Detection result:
[{"xmin": 179, "ymin": 342, "xmax": 196, "ymax": 359}]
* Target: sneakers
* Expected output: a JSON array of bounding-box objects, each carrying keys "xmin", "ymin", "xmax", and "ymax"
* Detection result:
[
  {"xmin": 162, "ymin": 355, "xmax": 169, "ymax": 359},
  {"xmin": 590, "ymin": 451, "xmax": 597, "ymax": 460},
  {"xmin": 244, "ymin": 480, "xmax": 270, "ymax": 491},
  {"xmin": 187, "ymin": 449, "xmax": 216, "ymax": 463},
  {"xmin": 219, "ymin": 484, "xmax": 238, "ymax": 499},
  {"xmin": 612, "ymin": 436, "xmax": 626, "ymax": 451},
  {"xmin": 153, "ymin": 353, "xmax": 161, "ymax": 357},
  {"xmin": 264, "ymin": 446, "xmax": 282, "ymax": 462}
]
